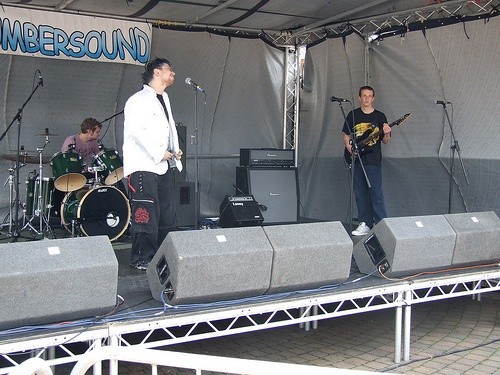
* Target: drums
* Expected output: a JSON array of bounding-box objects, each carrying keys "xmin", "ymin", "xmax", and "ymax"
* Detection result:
[
  {"xmin": 49, "ymin": 150, "xmax": 88, "ymax": 192},
  {"xmin": 93, "ymin": 150, "xmax": 123, "ymax": 186},
  {"xmin": 60, "ymin": 186, "xmax": 131, "ymax": 242},
  {"xmin": 25, "ymin": 176, "xmax": 65, "ymax": 219}
]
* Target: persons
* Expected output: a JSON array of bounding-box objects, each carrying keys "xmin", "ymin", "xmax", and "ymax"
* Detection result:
[
  {"xmin": 122, "ymin": 57, "xmax": 182, "ymax": 272},
  {"xmin": 61, "ymin": 118, "xmax": 128, "ymax": 237},
  {"xmin": 343, "ymin": 86, "xmax": 391, "ymax": 235}
]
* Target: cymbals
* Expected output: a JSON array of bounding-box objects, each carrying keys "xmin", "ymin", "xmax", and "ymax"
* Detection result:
[
  {"xmin": 1, "ymin": 154, "xmax": 48, "ymax": 164},
  {"xmin": 8, "ymin": 150, "xmax": 38, "ymax": 153},
  {"xmin": 34, "ymin": 133, "xmax": 61, "ymax": 138}
]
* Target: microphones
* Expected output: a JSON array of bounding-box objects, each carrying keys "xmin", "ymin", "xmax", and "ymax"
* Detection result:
[
  {"xmin": 185, "ymin": 77, "xmax": 205, "ymax": 92},
  {"xmin": 435, "ymin": 100, "xmax": 451, "ymax": 104},
  {"xmin": 38, "ymin": 71, "xmax": 43, "ymax": 86},
  {"xmin": 331, "ymin": 96, "xmax": 350, "ymax": 102},
  {"xmin": 45, "ymin": 128, "xmax": 50, "ymax": 143}
]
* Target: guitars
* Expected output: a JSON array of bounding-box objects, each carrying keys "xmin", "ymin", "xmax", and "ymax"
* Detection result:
[{"xmin": 343, "ymin": 112, "xmax": 413, "ymax": 165}]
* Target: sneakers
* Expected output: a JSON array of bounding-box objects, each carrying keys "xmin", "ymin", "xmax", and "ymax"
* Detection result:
[
  {"xmin": 352, "ymin": 222, "xmax": 370, "ymax": 236},
  {"xmin": 130, "ymin": 260, "xmax": 149, "ymax": 269}
]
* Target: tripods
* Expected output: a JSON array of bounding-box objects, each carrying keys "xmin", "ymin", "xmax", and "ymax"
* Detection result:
[
  {"xmin": 0, "ymin": 161, "xmax": 24, "ymax": 236},
  {"xmin": 19, "ymin": 137, "xmax": 58, "ymax": 236}
]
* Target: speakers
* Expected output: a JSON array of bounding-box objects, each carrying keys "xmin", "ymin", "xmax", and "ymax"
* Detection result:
[
  {"xmin": 262, "ymin": 220, "xmax": 354, "ymax": 294},
  {"xmin": 444, "ymin": 211, "xmax": 500, "ymax": 268},
  {"xmin": 235, "ymin": 166, "xmax": 300, "ymax": 224},
  {"xmin": 220, "ymin": 195, "xmax": 264, "ymax": 229},
  {"xmin": 353, "ymin": 214, "xmax": 457, "ymax": 278},
  {"xmin": 174, "ymin": 182, "xmax": 200, "ymax": 227},
  {"xmin": 0, "ymin": 235, "xmax": 119, "ymax": 331},
  {"xmin": 171, "ymin": 126, "xmax": 186, "ymax": 182},
  {"xmin": 146, "ymin": 225, "xmax": 273, "ymax": 305}
]
